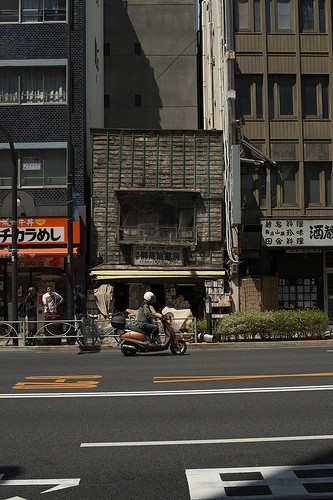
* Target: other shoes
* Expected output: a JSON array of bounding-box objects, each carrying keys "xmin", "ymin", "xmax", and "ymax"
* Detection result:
[{"xmin": 152, "ymin": 340, "xmax": 161, "ymax": 347}]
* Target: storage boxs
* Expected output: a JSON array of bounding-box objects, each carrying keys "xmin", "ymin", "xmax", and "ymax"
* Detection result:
[{"xmin": 110, "ymin": 315, "xmax": 127, "ymax": 329}]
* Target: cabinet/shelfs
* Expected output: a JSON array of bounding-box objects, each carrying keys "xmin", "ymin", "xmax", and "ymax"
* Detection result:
[{"xmin": 205, "ymin": 279, "xmax": 226, "ymax": 307}]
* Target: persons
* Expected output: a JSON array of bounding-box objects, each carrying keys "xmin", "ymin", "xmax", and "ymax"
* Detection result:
[
  {"xmin": 42, "ymin": 286, "xmax": 64, "ymax": 317},
  {"xmin": 133, "ymin": 292, "xmax": 167, "ymax": 345},
  {"xmin": 25, "ymin": 287, "xmax": 37, "ymax": 346},
  {"xmin": 73, "ymin": 285, "xmax": 88, "ymax": 344}
]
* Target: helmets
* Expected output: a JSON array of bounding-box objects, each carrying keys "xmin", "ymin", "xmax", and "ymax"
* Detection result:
[{"xmin": 144, "ymin": 292, "xmax": 156, "ymax": 304}]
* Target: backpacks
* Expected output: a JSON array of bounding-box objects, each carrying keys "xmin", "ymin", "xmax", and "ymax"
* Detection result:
[{"xmin": 18, "ymin": 303, "xmax": 26, "ymax": 317}]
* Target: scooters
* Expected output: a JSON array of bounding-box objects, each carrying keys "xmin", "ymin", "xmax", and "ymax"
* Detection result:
[{"xmin": 110, "ymin": 313, "xmax": 188, "ymax": 356}]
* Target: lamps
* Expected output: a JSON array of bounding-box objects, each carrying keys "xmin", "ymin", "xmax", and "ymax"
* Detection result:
[{"xmin": 17, "ymin": 195, "xmax": 26, "ymax": 217}]
